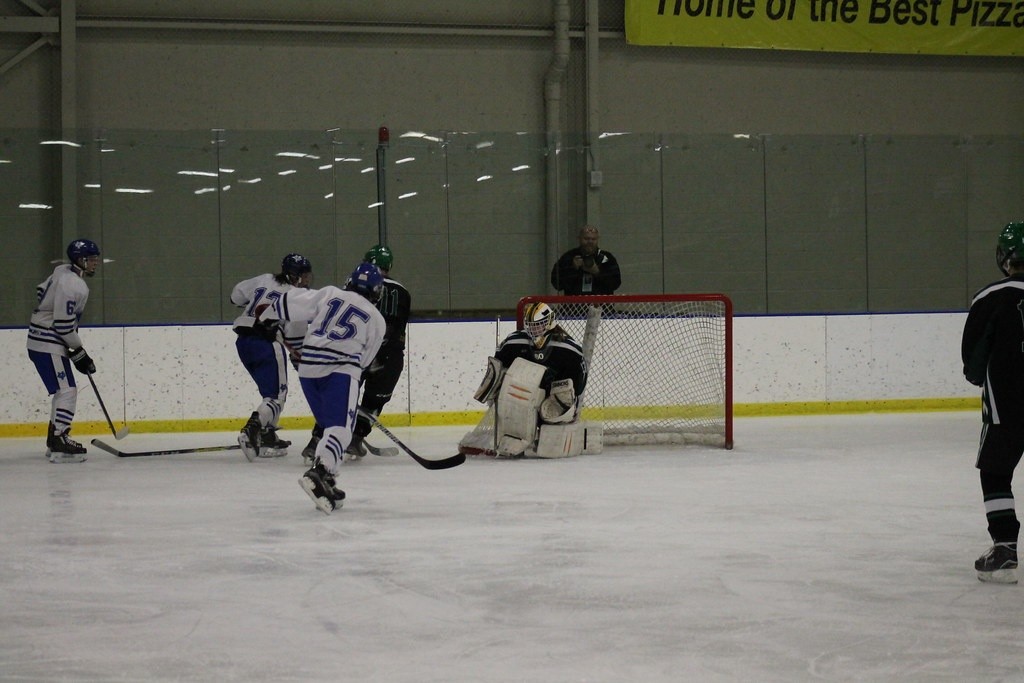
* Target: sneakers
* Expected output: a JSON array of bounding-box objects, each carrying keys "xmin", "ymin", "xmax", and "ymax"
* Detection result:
[
  {"xmin": 343, "ymin": 433, "xmax": 367, "ymax": 460},
  {"xmin": 238, "ymin": 411, "xmax": 263, "ymax": 461},
  {"xmin": 298, "ymin": 456, "xmax": 345, "ymax": 515},
  {"xmin": 975, "ymin": 539, "xmax": 1018, "ymax": 584},
  {"xmin": 259, "ymin": 421, "xmax": 291, "ymax": 457},
  {"xmin": 44, "ymin": 420, "xmax": 88, "ymax": 464},
  {"xmin": 301, "ymin": 424, "xmax": 324, "ymax": 466}
]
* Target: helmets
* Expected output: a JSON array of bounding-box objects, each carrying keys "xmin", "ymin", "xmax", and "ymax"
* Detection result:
[
  {"xmin": 281, "ymin": 253, "xmax": 314, "ymax": 288},
  {"xmin": 361, "ymin": 245, "xmax": 393, "ymax": 271},
  {"xmin": 524, "ymin": 301, "xmax": 560, "ymax": 350},
  {"xmin": 996, "ymin": 221, "xmax": 1024, "ymax": 276},
  {"xmin": 66, "ymin": 239, "xmax": 100, "ymax": 277},
  {"xmin": 343, "ymin": 264, "xmax": 385, "ymax": 303}
]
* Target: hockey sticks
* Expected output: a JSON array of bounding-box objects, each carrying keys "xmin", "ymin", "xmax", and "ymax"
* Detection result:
[
  {"xmin": 492, "ymin": 315, "xmax": 525, "ymax": 459},
  {"xmin": 354, "ymin": 432, "xmax": 399, "ymax": 458},
  {"xmin": 87, "ymin": 370, "xmax": 130, "ymax": 440},
  {"xmin": 91, "ymin": 438, "xmax": 291, "ymax": 457},
  {"xmin": 275, "ymin": 335, "xmax": 466, "ymax": 470}
]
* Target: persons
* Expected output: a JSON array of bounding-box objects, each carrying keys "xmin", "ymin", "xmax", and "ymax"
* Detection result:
[
  {"xmin": 551, "ymin": 225, "xmax": 622, "ymax": 312},
  {"xmin": 261, "ymin": 263, "xmax": 386, "ymax": 515},
  {"xmin": 230, "ymin": 254, "xmax": 312, "ymax": 463},
  {"xmin": 300, "ymin": 244, "xmax": 412, "ymax": 467},
  {"xmin": 26, "ymin": 239, "xmax": 100, "ymax": 463},
  {"xmin": 961, "ymin": 221, "xmax": 1024, "ymax": 584},
  {"xmin": 457, "ymin": 301, "xmax": 603, "ymax": 458}
]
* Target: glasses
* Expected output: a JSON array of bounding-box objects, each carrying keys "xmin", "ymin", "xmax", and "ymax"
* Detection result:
[{"xmin": 580, "ymin": 228, "xmax": 599, "ymax": 236}]
